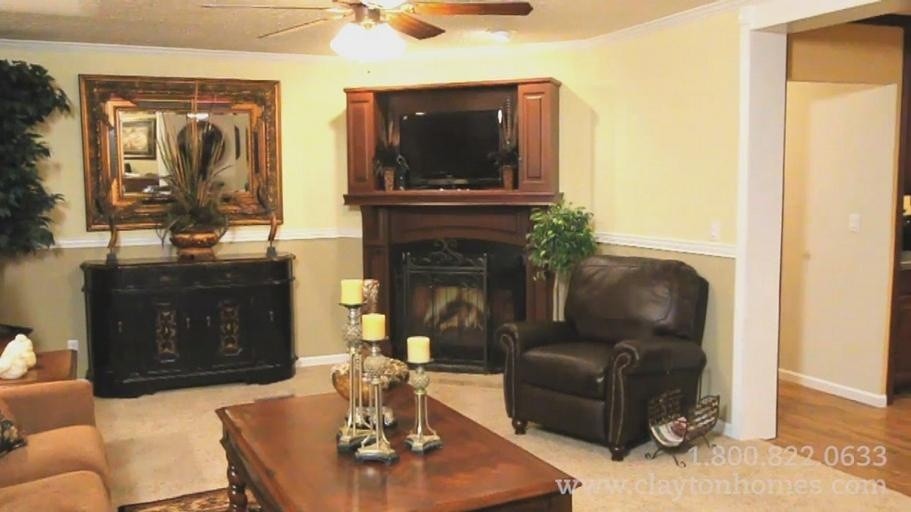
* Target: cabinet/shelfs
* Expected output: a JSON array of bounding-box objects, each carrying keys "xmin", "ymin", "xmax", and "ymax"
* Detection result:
[
  {"xmin": 78, "ymin": 250, "xmax": 298, "ymax": 398},
  {"xmin": 341, "ymin": 77, "xmax": 561, "ymax": 207}
]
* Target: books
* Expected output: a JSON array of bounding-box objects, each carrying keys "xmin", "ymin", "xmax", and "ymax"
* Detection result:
[{"xmin": 651, "ymin": 414, "xmax": 698, "ymax": 448}]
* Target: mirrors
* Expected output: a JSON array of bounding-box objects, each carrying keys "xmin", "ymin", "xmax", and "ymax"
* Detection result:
[{"xmin": 76, "ymin": 72, "xmax": 283, "ymax": 232}]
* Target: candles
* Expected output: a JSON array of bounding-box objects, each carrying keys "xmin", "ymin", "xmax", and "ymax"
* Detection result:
[
  {"xmin": 406, "ymin": 337, "xmax": 430, "ymax": 363},
  {"xmin": 340, "ymin": 279, "xmax": 363, "ymax": 305},
  {"xmin": 360, "ymin": 313, "xmax": 385, "ymax": 341}
]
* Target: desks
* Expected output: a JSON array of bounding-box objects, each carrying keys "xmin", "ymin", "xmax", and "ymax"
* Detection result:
[{"xmin": 0, "ymin": 349, "xmax": 77, "ymax": 385}]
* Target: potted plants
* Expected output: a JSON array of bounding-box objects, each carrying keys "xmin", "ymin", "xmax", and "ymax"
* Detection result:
[
  {"xmin": 150, "ymin": 79, "xmax": 236, "ymax": 259},
  {"xmin": 0, "ymin": 54, "xmax": 72, "ymax": 352}
]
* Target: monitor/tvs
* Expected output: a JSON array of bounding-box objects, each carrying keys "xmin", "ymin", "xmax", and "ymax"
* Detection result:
[{"xmin": 399, "ymin": 109, "xmax": 504, "ymax": 187}]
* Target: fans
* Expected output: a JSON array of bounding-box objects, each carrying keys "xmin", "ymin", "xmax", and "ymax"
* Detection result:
[{"xmin": 198, "ymin": 0, "xmax": 532, "ymax": 41}]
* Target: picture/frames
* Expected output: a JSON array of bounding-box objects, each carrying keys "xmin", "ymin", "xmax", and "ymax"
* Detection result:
[{"xmin": 120, "ymin": 117, "xmax": 155, "ymax": 159}]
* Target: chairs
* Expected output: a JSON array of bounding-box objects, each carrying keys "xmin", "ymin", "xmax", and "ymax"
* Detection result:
[{"xmin": 493, "ymin": 255, "xmax": 709, "ymax": 461}]
